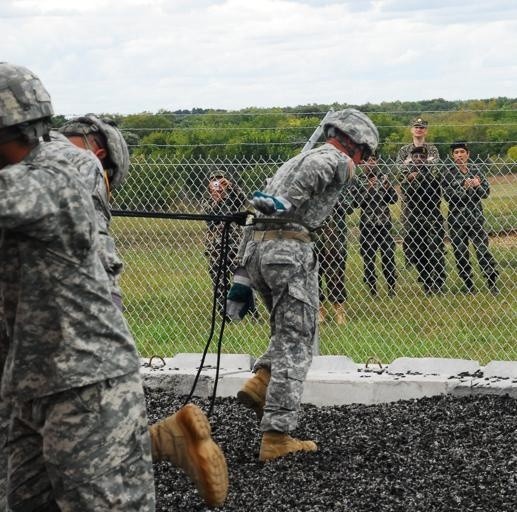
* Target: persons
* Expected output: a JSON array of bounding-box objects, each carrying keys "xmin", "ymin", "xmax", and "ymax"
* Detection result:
[
  {"xmin": 0, "ymin": 63, "xmax": 230, "ymax": 512},
  {"xmin": 396, "ymin": 117, "xmax": 444, "ymax": 270},
  {"xmin": 399, "ymin": 147, "xmax": 445, "ymax": 296},
  {"xmin": 355, "ymin": 150, "xmax": 399, "ymax": 298},
  {"xmin": 58, "ymin": 116, "xmax": 130, "ymax": 190},
  {"xmin": 220, "ymin": 105, "xmax": 380, "ymax": 464},
  {"xmin": 203, "ymin": 169, "xmax": 248, "ymax": 323},
  {"xmin": 310, "ymin": 188, "xmax": 355, "ymax": 324},
  {"xmin": 443, "ymin": 143, "xmax": 497, "ymax": 293}
]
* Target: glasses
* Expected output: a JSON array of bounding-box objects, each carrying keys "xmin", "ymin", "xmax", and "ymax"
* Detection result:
[{"xmin": 359, "ymin": 146, "xmax": 373, "ymax": 161}]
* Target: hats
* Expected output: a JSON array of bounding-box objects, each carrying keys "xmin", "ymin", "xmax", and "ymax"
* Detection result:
[
  {"xmin": 450, "ymin": 140, "xmax": 468, "ymax": 153},
  {"xmin": 412, "ymin": 147, "xmax": 428, "ymax": 157},
  {"xmin": 209, "ymin": 170, "xmax": 228, "ymax": 178},
  {"xmin": 410, "ymin": 116, "xmax": 428, "ymax": 128}
]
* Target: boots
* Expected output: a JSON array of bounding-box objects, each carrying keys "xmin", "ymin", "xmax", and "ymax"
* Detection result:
[
  {"xmin": 425, "ymin": 280, "xmax": 444, "ymax": 293},
  {"xmin": 238, "ymin": 367, "xmax": 271, "ymax": 420},
  {"xmin": 463, "ymin": 276, "xmax": 475, "ymax": 292},
  {"xmin": 387, "ymin": 283, "xmax": 396, "ymax": 296},
  {"xmin": 487, "ymin": 273, "xmax": 499, "ymax": 294},
  {"xmin": 319, "ymin": 302, "xmax": 326, "ymax": 322},
  {"xmin": 369, "ymin": 281, "xmax": 378, "ymax": 295},
  {"xmin": 334, "ymin": 302, "xmax": 348, "ymax": 324},
  {"xmin": 259, "ymin": 428, "xmax": 319, "ymax": 463},
  {"xmin": 148, "ymin": 403, "xmax": 229, "ymax": 508}
]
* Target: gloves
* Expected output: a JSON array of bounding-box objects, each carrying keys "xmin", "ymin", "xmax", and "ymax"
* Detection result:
[
  {"xmin": 250, "ymin": 191, "xmax": 293, "ymax": 216},
  {"xmin": 220, "ymin": 274, "xmax": 261, "ymax": 321}
]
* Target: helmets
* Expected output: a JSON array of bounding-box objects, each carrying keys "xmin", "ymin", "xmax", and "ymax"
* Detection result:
[
  {"xmin": 84, "ymin": 112, "xmax": 130, "ymax": 190},
  {"xmin": 0, "ymin": 61, "xmax": 55, "ymax": 127},
  {"xmin": 323, "ymin": 108, "xmax": 382, "ymax": 154}
]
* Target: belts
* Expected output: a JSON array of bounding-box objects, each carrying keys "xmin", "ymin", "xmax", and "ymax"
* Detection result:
[{"xmin": 252, "ymin": 229, "xmax": 311, "ymax": 241}]
